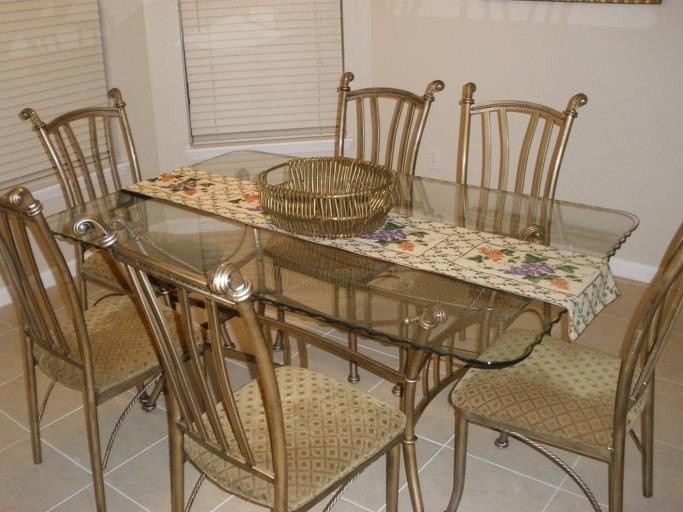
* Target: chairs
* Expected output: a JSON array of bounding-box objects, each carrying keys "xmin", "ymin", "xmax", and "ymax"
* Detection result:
[
  {"xmin": 73, "ymin": 215, "xmax": 407, "ymax": 512},
  {"xmin": 444, "ymin": 220, "xmax": 683, "ymax": 512},
  {"xmin": 392, "ymin": 81, "xmax": 588, "ymax": 396},
  {"xmin": 272, "ymin": 72, "xmax": 445, "ymax": 383},
  {"xmin": 21, "ymin": 87, "xmax": 235, "ymax": 402},
  {"xmin": 1, "ymin": 187, "xmax": 226, "ymax": 512}
]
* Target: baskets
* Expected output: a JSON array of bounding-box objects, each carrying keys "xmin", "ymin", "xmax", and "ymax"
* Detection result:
[{"xmin": 255, "ymin": 155, "xmax": 401, "ymax": 238}]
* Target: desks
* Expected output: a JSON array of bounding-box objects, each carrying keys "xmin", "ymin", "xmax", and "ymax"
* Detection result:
[{"xmin": 43, "ymin": 149, "xmax": 639, "ymax": 512}]
